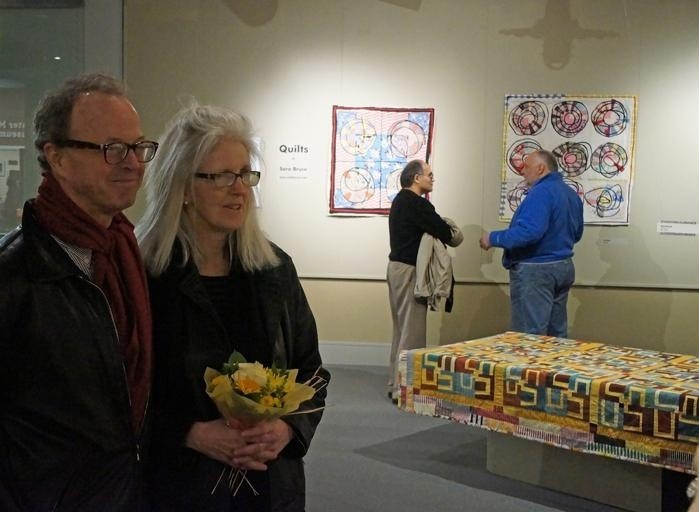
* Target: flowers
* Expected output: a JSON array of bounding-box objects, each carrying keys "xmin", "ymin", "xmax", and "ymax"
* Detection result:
[{"xmin": 204, "ymin": 350, "xmax": 316, "ymax": 497}]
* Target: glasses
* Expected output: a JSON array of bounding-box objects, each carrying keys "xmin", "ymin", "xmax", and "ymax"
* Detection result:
[
  {"xmin": 39, "ymin": 137, "xmax": 159, "ymax": 165},
  {"xmin": 193, "ymin": 171, "xmax": 262, "ymax": 190}
]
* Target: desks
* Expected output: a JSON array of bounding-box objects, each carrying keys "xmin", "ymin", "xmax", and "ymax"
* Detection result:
[{"xmin": 397, "ymin": 331, "xmax": 699, "ymax": 512}]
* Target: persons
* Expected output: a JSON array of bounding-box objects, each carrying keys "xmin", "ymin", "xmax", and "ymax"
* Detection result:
[
  {"xmin": 477, "ymin": 151, "xmax": 583, "ymax": 337},
  {"xmin": 134, "ymin": 105, "xmax": 326, "ymax": 512},
  {"xmin": 387, "ymin": 160, "xmax": 465, "ymax": 404},
  {"xmin": 0, "ymin": 74, "xmax": 158, "ymax": 511}
]
